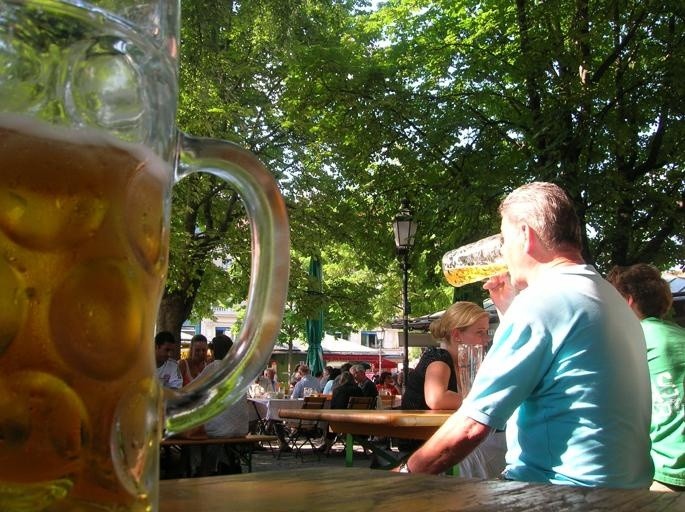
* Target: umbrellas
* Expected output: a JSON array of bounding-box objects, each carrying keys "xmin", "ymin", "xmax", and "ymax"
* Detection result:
[{"xmin": 303, "ymin": 253, "xmax": 326, "ymax": 376}]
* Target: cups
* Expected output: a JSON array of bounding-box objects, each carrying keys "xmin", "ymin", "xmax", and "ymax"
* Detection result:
[
  {"xmin": 0, "ymin": 1, "xmax": 289, "ymax": 511},
  {"xmin": 303, "ymin": 387, "xmax": 318, "ymax": 397},
  {"xmin": 459, "ymin": 343, "xmax": 483, "ymax": 404},
  {"xmin": 440, "ymin": 235, "xmax": 509, "ymax": 287}
]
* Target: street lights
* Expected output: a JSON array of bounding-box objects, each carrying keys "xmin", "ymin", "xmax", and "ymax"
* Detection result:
[
  {"xmin": 391, "ymin": 209, "xmax": 420, "ymax": 386},
  {"xmin": 391, "ymin": 197, "xmax": 418, "ymax": 394},
  {"xmin": 374, "ymin": 323, "xmax": 385, "ymax": 378}
]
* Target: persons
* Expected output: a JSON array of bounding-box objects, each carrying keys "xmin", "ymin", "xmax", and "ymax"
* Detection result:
[
  {"xmin": 203, "ymin": 334, "xmax": 251, "ymax": 438},
  {"xmin": 180, "ymin": 333, "xmax": 209, "ymax": 386},
  {"xmin": 602, "ymin": 263, "xmax": 684, "ymax": 493},
  {"xmin": 153, "ymin": 330, "xmax": 183, "ymax": 390},
  {"xmin": 393, "ymin": 181, "xmax": 656, "ymax": 491},
  {"xmin": 248, "ymin": 357, "xmax": 403, "ymax": 452},
  {"xmin": 398, "ymin": 300, "xmax": 491, "ymax": 455}
]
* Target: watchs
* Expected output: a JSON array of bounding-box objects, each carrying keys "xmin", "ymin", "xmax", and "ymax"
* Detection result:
[{"xmin": 398, "ymin": 458, "xmax": 410, "ymax": 475}]
